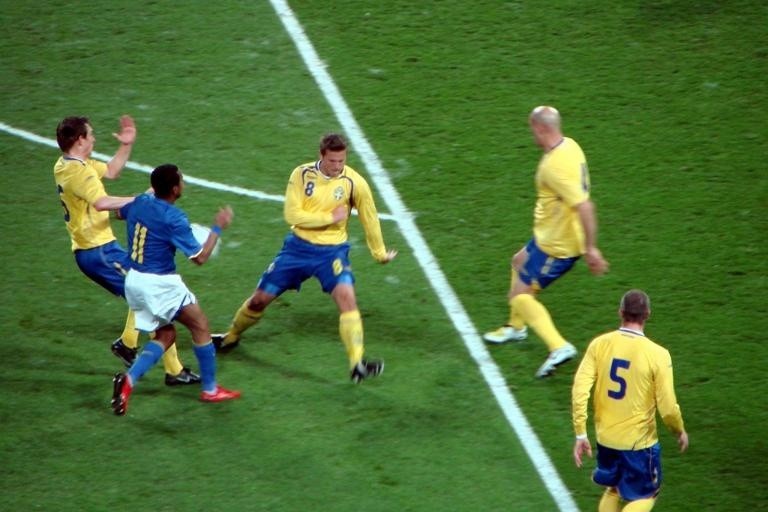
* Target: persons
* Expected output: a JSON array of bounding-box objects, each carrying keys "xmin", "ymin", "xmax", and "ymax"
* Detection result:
[
  {"xmin": 109, "ymin": 165, "xmax": 240, "ymax": 415},
  {"xmin": 210, "ymin": 134, "xmax": 398, "ymax": 386},
  {"xmin": 480, "ymin": 106, "xmax": 611, "ymax": 380},
  {"xmin": 53, "ymin": 113, "xmax": 200, "ymax": 386},
  {"xmin": 573, "ymin": 290, "xmax": 689, "ymax": 512}
]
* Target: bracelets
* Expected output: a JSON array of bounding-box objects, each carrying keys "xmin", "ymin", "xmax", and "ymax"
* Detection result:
[
  {"xmin": 576, "ymin": 433, "xmax": 587, "ymax": 440},
  {"xmin": 210, "ymin": 225, "xmax": 219, "ymax": 235}
]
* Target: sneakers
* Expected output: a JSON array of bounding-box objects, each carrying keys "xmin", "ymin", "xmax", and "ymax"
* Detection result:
[
  {"xmin": 111, "ymin": 338, "xmax": 137, "ymax": 368},
  {"xmin": 351, "ymin": 359, "xmax": 384, "ymax": 385},
  {"xmin": 483, "ymin": 325, "xmax": 528, "ymax": 343},
  {"xmin": 199, "ymin": 384, "xmax": 241, "ymax": 403},
  {"xmin": 211, "ymin": 334, "xmax": 239, "ymax": 353},
  {"xmin": 165, "ymin": 368, "xmax": 201, "ymax": 386},
  {"xmin": 111, "ymin": 372, "xmax": 132, "ymax": 416},
  {"xmin": 535, "ymin": 343, "xmax": 578, "ymax": 379}
]
